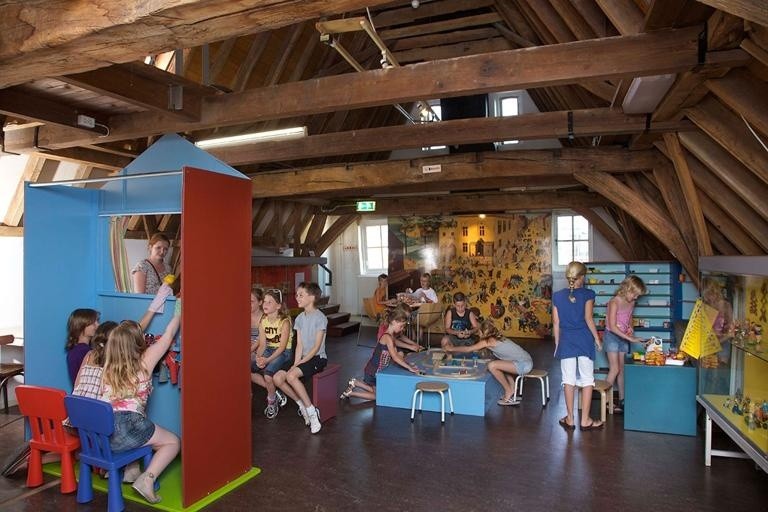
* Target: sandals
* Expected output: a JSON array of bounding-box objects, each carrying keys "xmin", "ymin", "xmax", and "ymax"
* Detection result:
[
  {"xmin": 605, "ymin": 402, "xmax": 624, "ymax": 415},
  {"xmin": 122, "ymin": 468, "xmax": 146, "ymax": 484},
  {"xmin": 131, "ymin": 472, "xmax": 162, "ymax": 505},
  {"xmin": 617, "ymin": 399, "xmax": 624, "ymax": 410}
]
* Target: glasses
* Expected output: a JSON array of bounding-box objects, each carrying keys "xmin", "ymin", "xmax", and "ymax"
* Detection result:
[{"xmin": 264, "ymin": 287, "xmax": 284, "ymax": 303}]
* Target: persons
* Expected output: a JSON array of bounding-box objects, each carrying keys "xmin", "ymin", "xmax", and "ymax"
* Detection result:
[
  {"xmin": 250, "ymin": 288, "xmax": 294, "ymax": 420},
  {"xmin": 552, "ymin": 261, "xmax": 604, "ymax": 430},
  {"xmin": 251, "ymin": 286, "xmax": 267, "ymax": 362},
  {"xmin": 699, "ymin": 277, "xmax": 734, "ymax": 356},
  {"xmin": 272, "ymin": 281, "xmax": 329, "ymax": 434},
  {"xmin": 440, "ymin": 292, "xmax": 479, "ymax": 347},
  {"xmin": 339, "ymin": 309, "xmax": 418, "ymax": 401},
  {"xmin": 63, "ymin": 308, "xmax": 102, "ymax": 382},
  {"xmin": 97, "ymin": 296, "xmax": 182, "ymax": 503},
  {"xmin": 601, "ymin": 275, "xmax": 647, "ymax": 414},
  {"xmin": 372, "ymin": 274, "xmax": 397, "ymax": 320},
  {"xmin": 409, "ymin": 272, "xmax": 438, "ymax": 344},
  {"xmin": 376, "ymin": 302, "xmax": 425, "ymax": 357},
  {"xmin": 61, "ymin": 283, "xmax": 173, "ymax": 478},
  {"xmin": 446, "ymin": 323, "xmax": 533, "ymax": 406},
  {"xmin": 131, "ymin": 232, "xmax": 172, "ymax": 295}
]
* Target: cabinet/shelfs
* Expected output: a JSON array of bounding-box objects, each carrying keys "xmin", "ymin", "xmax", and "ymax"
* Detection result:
[
  {"xmin": 696, "ymin": 255, "xmax": 768, "ymax": 474},
  {"xmin": 582, "ymin": 260, "xmax": 701, "ymax": 400}
]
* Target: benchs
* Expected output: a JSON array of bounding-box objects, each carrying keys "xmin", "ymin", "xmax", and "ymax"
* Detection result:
[{"xmin": 290, "ymin": 356, "xmax": 341, "ymax": 424}]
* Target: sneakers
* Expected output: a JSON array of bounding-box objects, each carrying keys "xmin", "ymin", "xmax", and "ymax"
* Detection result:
[
  {"xmin": 340, "ymin": 386, "xmax": 353, "ymax": 400},
  {"xmin": 274, "ymin": 388, "xmax": 288, "ymax": 407},
  {"xmin": 307, "ymin": 405, "xmax": 322, "ymax": 433},
  {"xmin": 347, "ymin": 376, "xmax": 357, "ymax": 389},
  {"xmin": 265, "ymin": 397, "xmax": 278, "ymax": 420},
  {"xmin": 297, "ymin": 404, "xmax": 311, "ymax": 427}
]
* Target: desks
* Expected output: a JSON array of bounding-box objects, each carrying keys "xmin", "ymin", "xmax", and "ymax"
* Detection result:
[
  {"xmin": 623, "ymin": 361, "xmax": 697, "ymax": 438},
  {"xmin": 386, "ymin": 302, "xmax": 422, "ymax": 341}
]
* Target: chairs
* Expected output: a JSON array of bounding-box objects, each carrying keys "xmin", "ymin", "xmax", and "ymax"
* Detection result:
[
  {"xmin": 64, "ymin": 394, "xmax": 160, "ymax": 512},
  {"xmin": 357, "ymin": 297, "xmax": 385, "ymax": 348},
  {"xmin": 14, "ymin": 386, "xmax": 101, "ymax": 494},
  {"xmin": 0, "ymin": 335, "xmax": 24, "ymax": 414},
  {"xmin": 409, "ymin": 303, "xmax": 444, "ymax": 345}
]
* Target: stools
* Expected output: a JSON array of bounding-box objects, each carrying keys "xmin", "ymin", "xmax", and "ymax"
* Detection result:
[
  {"xmin": 578, "ymin": 380, "xmax": 613, "ymax": 422},
  {"xmin": 411, "ymin": 381, "xmax": 454, "ymax": 424},
  {"xmin": 514, "ymin": 369, "xmax": 549, "ymax": 407}
]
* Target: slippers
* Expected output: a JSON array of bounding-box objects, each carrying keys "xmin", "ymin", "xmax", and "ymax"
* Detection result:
[
  {"xmin": 580, "ymin": 416, "xmax": 606, "ymax": 432},
  {"xmin": 498, "ymin": 393, "xmax": 523, "ymax": 407},
  {"xmin": 558, "ymin": 415, "xmax": 577, "ymax": 430}
]
